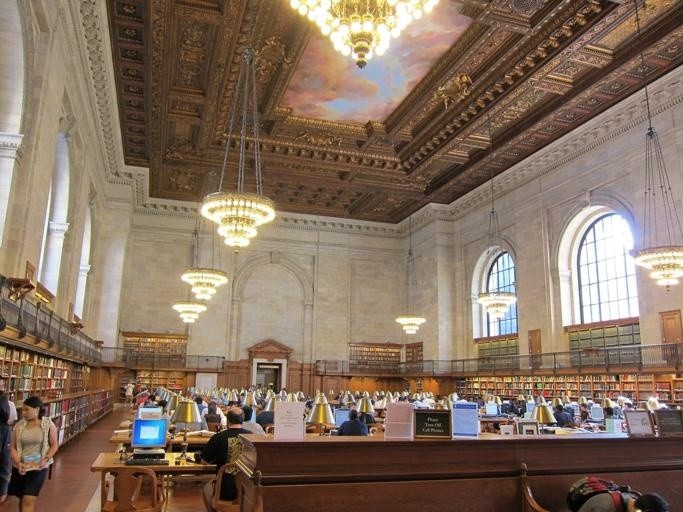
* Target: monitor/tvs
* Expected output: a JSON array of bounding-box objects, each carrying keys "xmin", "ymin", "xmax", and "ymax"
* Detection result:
[
  {"xmin": 503, "ymin": 400, "xmax": 509, "ymax": 404},
  {"xmin": 486, "ymin": 403, "xmax": 497, "ymax": 417},
  {"xmin": 526, "ymin": 403, "xmax": 536, "ymax": 413},
  {"xmin": 334, "ymin": 408, "xmax": 352, "ymax": 427},
  {"xmin": 137, "ymin": 406, "xmax": 163, "ymax": 418},
  {"xmin": 572, "ymin": 404, "xmax": 579, "ymax": 415},
  {"xmin": 592, "ymin": 407, "xmax": 603, "ymax": 421},
  {"xmin": 250, "ymin": 408, "xmax": 256, "ymax": 423},
  {"xmin": 131, "ymin": 418, "xmax": 169, "ymax": 450}
]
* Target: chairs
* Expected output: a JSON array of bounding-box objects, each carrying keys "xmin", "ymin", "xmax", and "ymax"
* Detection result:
[
  {"xmin": 102, "ymin": 467, "xmax": 166, "ymax": 511},
  {"xmin": 211, "ymin": 462, "xmax": 238, "ymax": 511}
]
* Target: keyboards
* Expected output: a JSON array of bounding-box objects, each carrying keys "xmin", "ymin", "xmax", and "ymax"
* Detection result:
[{"xmin": 125, "ymin": 459, "xmax": 169, "ymax": 465}]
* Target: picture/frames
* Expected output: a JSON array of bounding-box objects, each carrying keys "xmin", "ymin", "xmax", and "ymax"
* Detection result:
[{"xmin": 623, "ymin": 410, "xmax": 656, "ymax": 437}]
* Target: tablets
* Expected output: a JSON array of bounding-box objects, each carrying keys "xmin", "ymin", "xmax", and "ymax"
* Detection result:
[{"xmin": 451, "ymin": 402, "xmax": 479, "ymax": 439}]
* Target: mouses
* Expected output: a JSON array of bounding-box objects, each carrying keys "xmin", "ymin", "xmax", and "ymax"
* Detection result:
[
  {"xmin": 175, "ymin": 460, "xmax": 180, "ymax": 465},
  {"xmin": 171, "ymin": 435, "xmax": 174, "ymax": 439}
]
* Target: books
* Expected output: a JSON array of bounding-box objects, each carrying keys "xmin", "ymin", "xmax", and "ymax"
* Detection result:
[
  {"xmin": 454, "ymin": 374, "xmax": 682, "ymax": 407},
  {"xmin": 0, "ymin": 343, "xmax": 116, "ymax": 451}
]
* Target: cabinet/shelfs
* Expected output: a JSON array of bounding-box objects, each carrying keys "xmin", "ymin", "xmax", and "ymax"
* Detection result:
[
  {"xmin": 473, "ymin": 321, "xmax": 642, "ymax": 373},
  {"xmin": 455, "ymin": 374, "xmax": 682, "ymax": 411},
  {"xmin": 120, "ymin": 371, "xmax": 185, "ymax": 399},
  {"xmin": 237, "ymin": 434, "xmax": 678, "ymax": 512},
  {"xmin": 350, "ymin": 344, "xmax": 422, "ymax": 371},
  {"xmin": 0, "ymin": 336, "xmax": 115, "ymax": 450},
  {"xmin": 123, "ymin": 337, "xmax": 186, "ymax": 353}
]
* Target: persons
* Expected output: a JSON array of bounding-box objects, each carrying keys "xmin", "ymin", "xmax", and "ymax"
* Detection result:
[
  {"xmin": 9, "ymin": 396, "xmax": 59, "ymax": 511},
  {"xmin": 0, "ymin": 389, "xmax": 17, "ymax": 510},
  {"xmin": 122, "ymin": 379, "xmax": 681, "ymax": 511}
]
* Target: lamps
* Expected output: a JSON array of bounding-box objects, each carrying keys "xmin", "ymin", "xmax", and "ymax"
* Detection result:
[
  {"xmin": 200, "ymin": 50, "xmax": 275, "ymax": 258},
  {"xmin": 89, "ymin": 385, "xmax": 626, "ymax": 476},
  {"xmin": 285, "ymin": 2, "xmax": 442, "ymax": 68},
  {"xmin": 181, "ymin": 209, "xmax": 228, "ymax": 303},
  {"xmin": 478, "ymin": 114, "xmax": 518, "ymax": 323},
  {"xmin": 174, "ymin": 302, "xmax": 207, "ymax": 325},
  {"xmin": 7, "ymin": 278, "xmax": 35, "ymax": 302},
  {"xmin": 630, "ymin": 3, "xmax": 683, "ymax": 291},
  {"xmin": 396, "ymin": 183, "xmax": 427, "ymax": 337}
]
